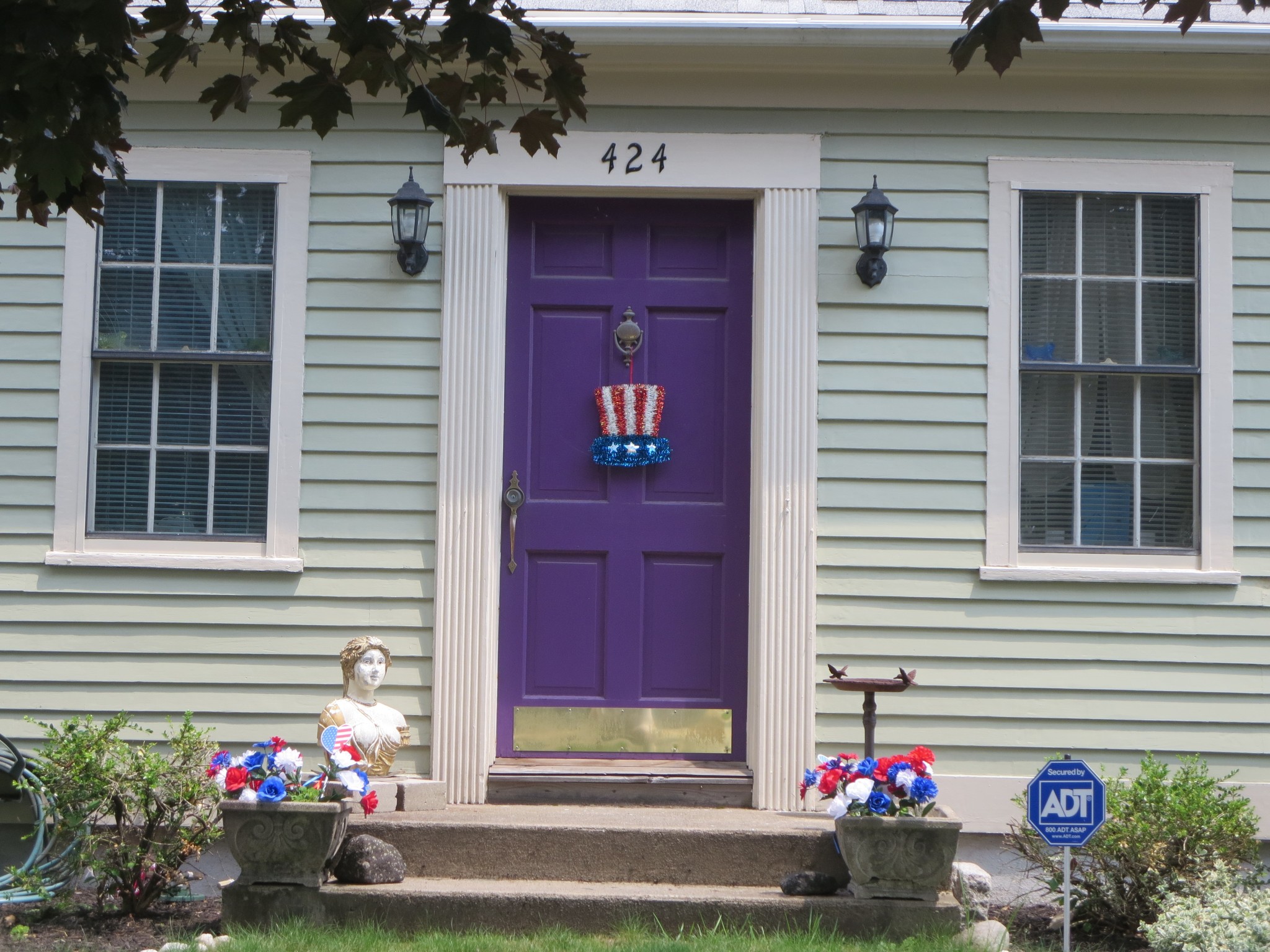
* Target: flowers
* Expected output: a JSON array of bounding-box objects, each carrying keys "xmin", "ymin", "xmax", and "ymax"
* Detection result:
[
  {"xmin": 797, "ymin": 744, "xmax": 939, "ymax": 821},
  {"xmin": 203, "ymin": 737, "xmax": 379, "ymax": 820}
]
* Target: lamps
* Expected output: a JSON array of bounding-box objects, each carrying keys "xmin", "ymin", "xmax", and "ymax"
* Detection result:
[
  {"xmin": 387, "ymin": 166, "xmax": 434, "ymax": 276},
  {"xmin": 851, "ymin": 175, "xmax": 899, "ymax": 288}
]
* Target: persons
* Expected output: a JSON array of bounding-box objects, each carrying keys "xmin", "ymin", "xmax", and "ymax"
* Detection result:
[{"xmin": 316, "ymin": 635, "xmax": 412, "ymax": 777}]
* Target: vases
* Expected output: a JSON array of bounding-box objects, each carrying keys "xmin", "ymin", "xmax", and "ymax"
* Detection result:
[
  {"xmin": 218, "ymin": 795, "xmax": 354, "ymax": 889},
  {"xmin": 833, "ymin": 803, "xmax": 963, "ymax": 902}
]
